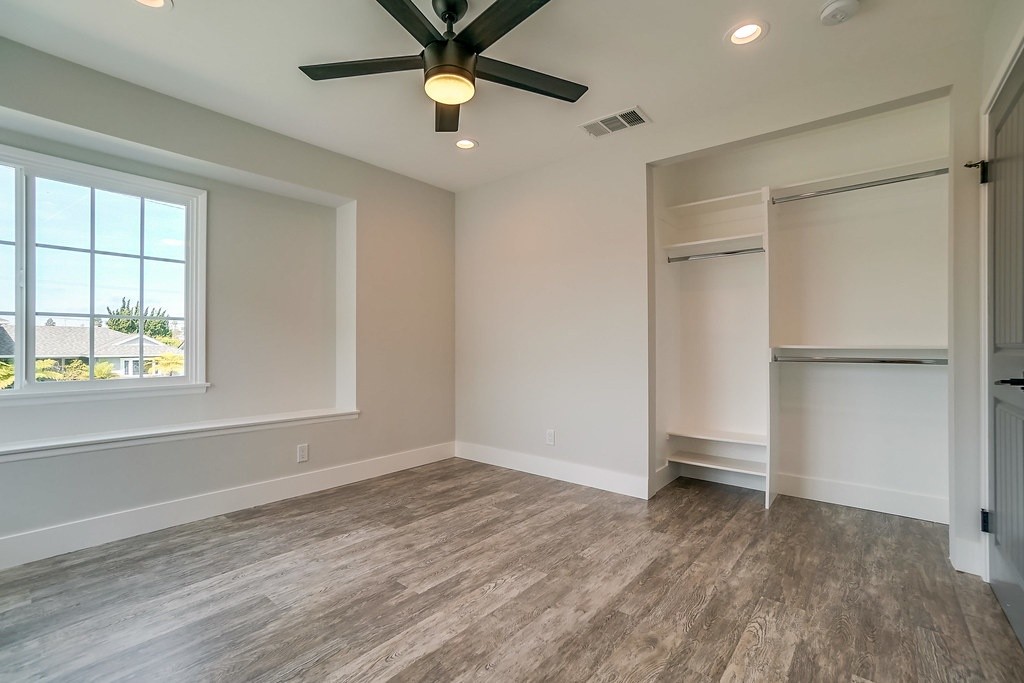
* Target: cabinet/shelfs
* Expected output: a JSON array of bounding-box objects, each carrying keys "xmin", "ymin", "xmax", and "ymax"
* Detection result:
[{"xmin": 661, "ymin": 160, "xmax": 950, "ymax": 527}]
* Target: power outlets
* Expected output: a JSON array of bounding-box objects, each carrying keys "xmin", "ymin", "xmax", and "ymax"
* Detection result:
[
  {"xmin": 546, "ymin": 429, "xmax": 555, "ymax": 445},
  {"xmin": 298, "ymin": 444, "xmax": 308, "ymax": 462}
]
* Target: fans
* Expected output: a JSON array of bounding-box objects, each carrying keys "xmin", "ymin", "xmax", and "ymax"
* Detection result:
[{"xmin": 297, "ymin": 0, "xmax": 589, "ymax": 132}]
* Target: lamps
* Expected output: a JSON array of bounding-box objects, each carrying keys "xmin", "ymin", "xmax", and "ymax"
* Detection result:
[{"xmin": 422, "ymin": 42, "xmax": 475, "ymax": 105}]
